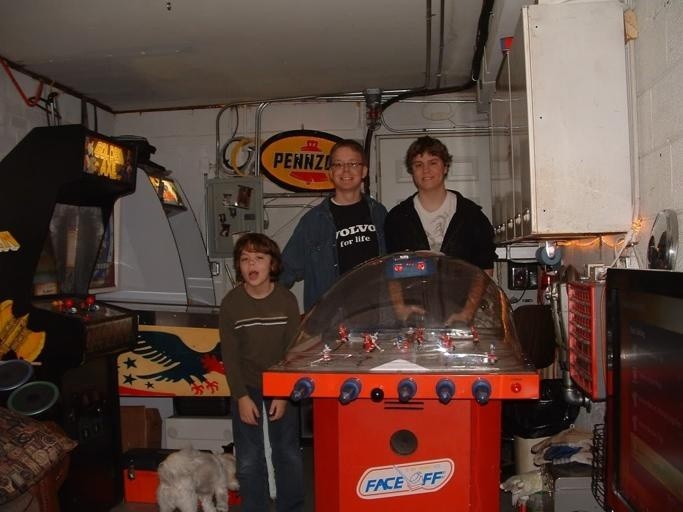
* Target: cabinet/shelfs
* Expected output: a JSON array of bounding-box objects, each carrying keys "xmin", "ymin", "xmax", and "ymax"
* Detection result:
[{"xmin": 486, "ymin": 2, "xmax": 634, "ymax": 247}]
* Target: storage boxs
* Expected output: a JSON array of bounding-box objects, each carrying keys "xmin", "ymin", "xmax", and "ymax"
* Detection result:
[{"xmin": 118, "ymin": 405, "xmax": 233, "ymax": 505}]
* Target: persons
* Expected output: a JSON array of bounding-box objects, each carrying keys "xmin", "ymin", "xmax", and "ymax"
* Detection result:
[
  {"xmin": 272, "ymin": 137, "xmax": 397, "ymax": 342},
  {"xmin": 385, "ymin": 134, "xmax": 495, "ymax": 328},
  {"xmin": 219, "ymin": 232, "xmax": 303, "ymax": 511}
]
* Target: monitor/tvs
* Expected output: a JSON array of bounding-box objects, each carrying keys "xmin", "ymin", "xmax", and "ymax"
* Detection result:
[
  {"xmin": 30, "ymin": 202, "xmax": 106, "ymax": 300},
  {"xmin": 601, "ymin": 268, "xmax": 683, "ymax": 512}
]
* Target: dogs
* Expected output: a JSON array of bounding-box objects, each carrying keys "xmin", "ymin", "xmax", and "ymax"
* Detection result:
[{"xmin": 156, "ymin": 443, "xmax": 240, "ymax": 512}]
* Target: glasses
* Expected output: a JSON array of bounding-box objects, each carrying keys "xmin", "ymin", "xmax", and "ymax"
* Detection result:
[{"xmin": 330, "ymin": 160, "xmax": 364, "ymax": 168}]
